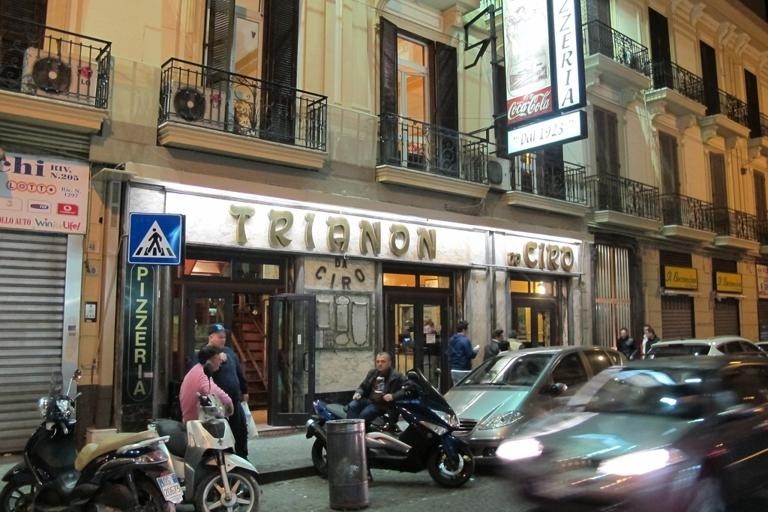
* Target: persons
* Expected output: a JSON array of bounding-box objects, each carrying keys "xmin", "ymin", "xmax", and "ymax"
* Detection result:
[
  {"xmin": 481, "ymin": 329, "xmax": 526, "ymax": 363},
  {"xmin": 616, "ymin": 324, "xmax": 663, "ymax": 361},
  {"xmin": 346, "ymin": 349, "xmax": 412, "ymax": 430},
  {"xmin": 422, "ymin": 320, "xmax": 440, "ymax": 388},
  {"xmin": 179, "ymin": 346, "xmax": 234, "ymax": 429},
  {"xmin": 204, "ymin": 324, "xmax": 263, "ymax": 500},
  {"xmin": 447, "ymin": 320, "xmax": 479, "ymax": 387}
]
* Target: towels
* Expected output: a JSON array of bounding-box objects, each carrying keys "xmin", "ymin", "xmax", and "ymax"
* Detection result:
[{"xmin": 326, "ymin": 419, "xmax": 369, "ymax": 510}]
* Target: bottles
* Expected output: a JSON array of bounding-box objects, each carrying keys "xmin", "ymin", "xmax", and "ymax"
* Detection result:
[{"xmin": 208, "ymin": 323, "xmax": 228, "ymax": 336}]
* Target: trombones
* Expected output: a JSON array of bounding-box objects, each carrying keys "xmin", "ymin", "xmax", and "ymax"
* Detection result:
[{"xmin": 127, "ymin": 211, "xmax": 187, "ymax": 269}]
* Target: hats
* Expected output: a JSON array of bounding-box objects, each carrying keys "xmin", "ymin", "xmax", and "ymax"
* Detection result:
[
  {"xmin": 640, "ymin": 333, "xmax": 767, "ymax": 362},
  {"xmin": 756, "ymin": 341, "xmax": 767, "ymax": 351},
  {"xmin": 487, "ymin": 350, "xmax": 766, "ymax": 512},
  {"xmin": 432, "ymin": 340, "xmax": 624, "ymax": 482}
]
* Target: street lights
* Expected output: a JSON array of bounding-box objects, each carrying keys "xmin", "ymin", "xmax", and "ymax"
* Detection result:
[{"xmin": 240, "ymin": 400, "xmax": 258, "ymax": 441}]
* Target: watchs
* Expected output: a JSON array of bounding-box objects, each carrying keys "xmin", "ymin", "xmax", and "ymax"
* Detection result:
[
  {"xmin": 304, "ymin": 368, "xmax": 482, "ymax": 490},
  {"xmin": 138, "ymin": 361, "xmax": 264, "ymax": 512},
  {"xmin": 0, "ymin": 367, "xmax": 187, "ymax": 512}
]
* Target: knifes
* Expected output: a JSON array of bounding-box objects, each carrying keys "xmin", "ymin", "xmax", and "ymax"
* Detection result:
[
  {"xmin": 18, "ymin": 44, "xmax": 101, "ymax": 107},
  {"xmin": 463, "ymin": 152, "xmax": 511, "ymax": 192},
  {"xmin": 161, "ymin": 78, "xmax": 228, "ymax": 132}
]
